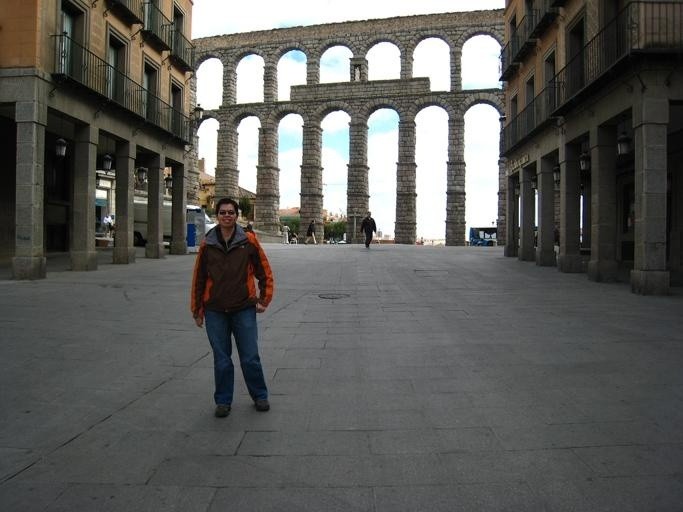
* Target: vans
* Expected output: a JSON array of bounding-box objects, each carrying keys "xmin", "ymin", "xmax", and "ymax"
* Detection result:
[{"xmin": 131, "ymin": 199, "xmax": 217, "ymax": 249}]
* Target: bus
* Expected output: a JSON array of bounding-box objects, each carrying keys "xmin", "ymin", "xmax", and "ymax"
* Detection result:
[{"xmin": 468, "ymin": 226, "xmax": 497, "ymax": 245}]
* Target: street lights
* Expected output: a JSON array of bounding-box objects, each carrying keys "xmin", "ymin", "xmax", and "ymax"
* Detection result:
[{"xmin": 348, "ymin": 207, "xmax": 358, "ymax": 243}]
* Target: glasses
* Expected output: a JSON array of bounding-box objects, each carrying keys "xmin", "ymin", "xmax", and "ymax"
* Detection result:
[{"xmin": 219, "ymin": 210, "xmax": 234, "ymax": 215}]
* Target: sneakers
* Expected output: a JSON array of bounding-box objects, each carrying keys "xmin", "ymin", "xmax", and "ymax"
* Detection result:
[
  {"xmin": 255, "ymin": 399, "xmax": 270, "ymax": 411},
  {"xmin": 215, "ymin": 405, "xmax": 231, "ymax": 417}
]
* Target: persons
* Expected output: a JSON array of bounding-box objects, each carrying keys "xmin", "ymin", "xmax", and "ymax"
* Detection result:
[
  {"xmin": 189, "ymin": 198, "xmax": 274, "ymax": 416},
  {"xmin": 103, "ymin": 212, "xmax": 112, "ymax": 237},
  {"xmin": 303, "ymin": 219, "xmax": 317, "ymax": 245},
  {"xmin": 360, "ymin": 211, "xmax": 376, "ymax": 248},
  {"xmin": 246, "ymin": 220, "xmax": 252, "ymax": 230},
  {"xmin": 281, "ymin": 223, "xmax": 290, "ymax": 243}
]
